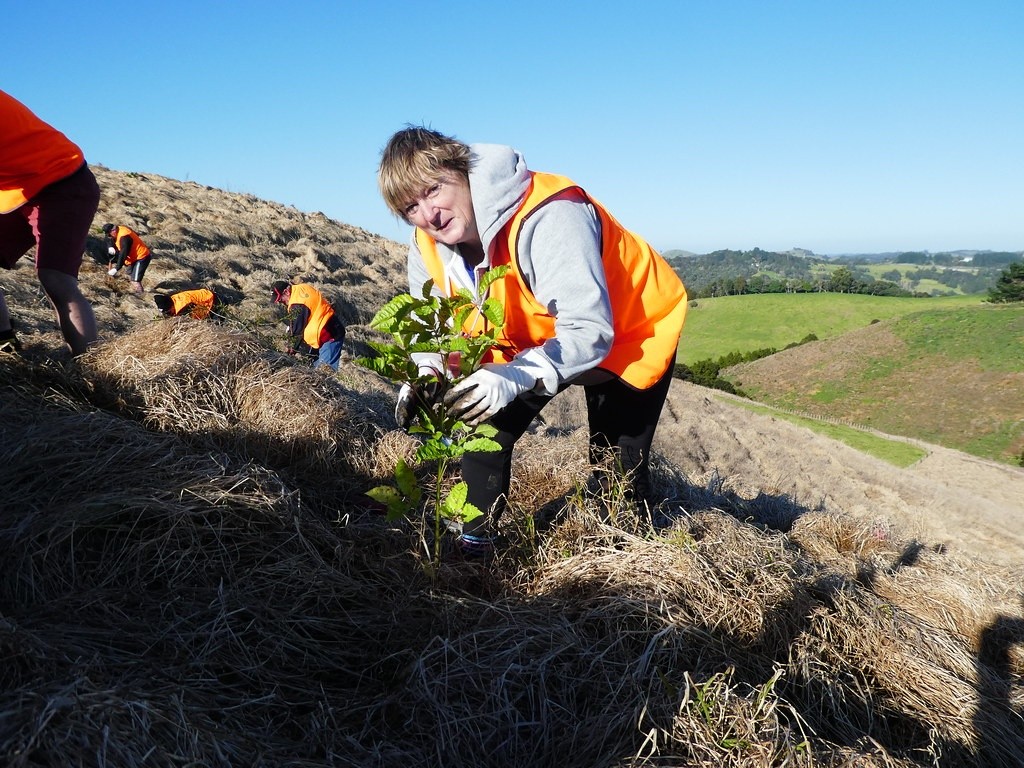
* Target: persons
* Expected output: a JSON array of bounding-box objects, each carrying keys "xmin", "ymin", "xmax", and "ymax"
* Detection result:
[
  {"xmin": 153, "ymin": 287, "xmax": 229, "ymax": 328},
  {"xmin": 269, "ymin": 281, "xmax": 345, "ymax": 372},
  {"xmin": 373, "ymin": 117, "xmax": 688, "ymax": 565},
  {"xmin": 102, "ymin": 222, "xmax": 152, "ymax": 293},
  {"xmin": 0, "ymin": 89, "xmax": 99, "ymax": 357}
]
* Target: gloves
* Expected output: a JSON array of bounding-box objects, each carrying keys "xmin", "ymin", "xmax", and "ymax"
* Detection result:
[
  {"xmin": 108, "ymin": 246, "xmax": 116, "ymax": 255},
  {"xmin": 107, "ymin": 268, "xmax": 117, "ymax": 276},
  {"xmin": 394, "ymin": 350, "xmax": 444, "ymax": 434},
  {"xmin": 442, "ymin": 347, "xmax": 561, "ymax": 427}
]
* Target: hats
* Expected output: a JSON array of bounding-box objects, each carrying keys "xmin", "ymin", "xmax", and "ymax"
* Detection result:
[
  {"xmin": 154, "ymin": 294, "xmax": 173, "ymax": 312},
  {"xmin": 270, "ymin": 280, "xmax": 290, "ymax": 304},
  {"xmin": 102, "ymin": 223, "xmax": 114, "ymax": 234}
]
